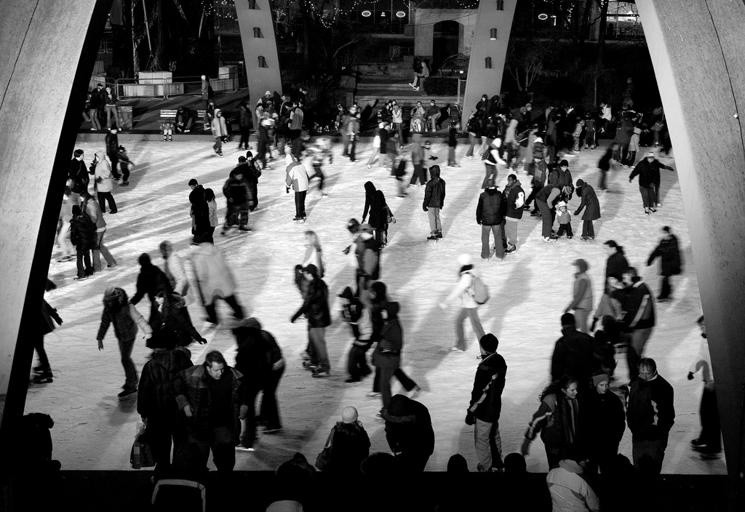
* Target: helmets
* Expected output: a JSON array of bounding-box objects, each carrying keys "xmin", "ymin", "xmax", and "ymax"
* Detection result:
[{"xmin": 346, "ymin": 218, "xmax": 359, "ymax": 232}]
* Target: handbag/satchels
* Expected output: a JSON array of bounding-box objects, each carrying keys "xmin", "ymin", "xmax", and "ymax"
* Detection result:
[
  {"xmin": 129, "ymin": 427, "xmax": 154, "ymax": 469},
  {"xmin": 315, "ymin": 445, "xmax": 332, "ymax": 471}
]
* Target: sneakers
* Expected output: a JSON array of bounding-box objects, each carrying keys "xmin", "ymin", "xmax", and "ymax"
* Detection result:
[
  {"xmin": 397, "ymin": 190, "xmax": 409, "ymax": 199},
  {"xmin": 341, "ymin": 153, "xmax": 359, "ymax": 164},
  {"xmin": 448, "ymin": 161, "xmax": 461, "ymax": 168},
  {"xmin": 560, "ymin": 145, "xmax": 599, "ymax": 153},
  {"xmin": 466, "ymin": 152, "xmax": 484, "ymax": 159},
  {"xmin": 163, "ymin": 137, "xmax": 173, "ymax": 142},
  {"xmin": 427, "ymin": 232, "xmax": 443, "ymax": 241},
  {"xmin": 100, "ymin": 208, "xmax": 118, "ymax": 214},
  {"xmin": 91, "ymin": 126, "xmax": 100, "ymax": 131},
  {"xmin": 58, "ymin": 254, "xmax": 74, "ymax": 264},
  {"xmin": 657, "ymin": 288, "xmax": 672, "ymax": 300},
  {"xmin": 522, "ymin": 204, "xmax": 542, "ymax": 219},
  {"xmin": 31, "ymin": 363, "xmax": 53, "ymax": 386},
  {"xmin": 259, "ymin": 152, "xmax": 286, "ymax": 170},
  {"xmin": 659, "ymin": 147, "xmax": 670, "ymax": 154},
  {"xmin": 343, "ymin": 367, "xmax": 421, "ymax": 422},
  {"xmin": 316, "ymin": 189, "xmax": 325, "ymax": 195},
  {"xmin": 73, "ymin": 260, "xmax": 117, "ymax": 280},
  {"xmin": 293, "ymin": 213, "xmax": 307, "ymax": 222},
  {"xmin": 301, "ymin": 349, "xmax": 331, "ymax": 379},
  {"xmin": 111, "ymin": 173, "xmax": 129, "ymax": 186},
  {"xmin": 481, "ymin": 242, "xmax": 516, "ymax": 259},
  {"xmin": 236, "ymin": 145, "xmax": 252, "ymax": 150},
  {"xmin": 117, "ymin": 376, "xmax": 140, "ymax": 400},
  {"xmin": 236, "ymin": 414, "xmax": 284, "ymax": 452},
  {"xmin": 645, "ymin": 202, "xmax": 662, "ymax": 216},
  {"xmin": 450, "ymin": 346, "xmax": 465, "ymax": 352},
  {"xmin": 212, "ymin": 145, "xmax": 224, "ymax": 156},
  {"xmin": 597, "ymin": 183, "xmax": 608, "ymax": 191},
  {"xmin": 220, "ymin": 222, "xmax": 253, "ymax": 236},
  {"xmin": 478, "ymin": 462, "xmax": 503, "ymax": 473},
  {"xmin": 543, "ymin": 231, "xmax": 594, "ymax": 242},
  {"xmin": 690, "ymin": 438, "xmax": 720, "ymax": 460},
  {"xmin": 408, "ymin": 180, "xmax": 428, "ymax": 188},
  {"xmin": 612, "ymin": 158, "xmax": 636, "ymax": 169},
  {"xmin": 365, "ymin": 162, "xmax": 394, "ymax": 175}
]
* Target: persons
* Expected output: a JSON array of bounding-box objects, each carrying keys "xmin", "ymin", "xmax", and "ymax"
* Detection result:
[{"xmin": 20, "ymin": 59, "xmax": 723, "ymax": 512}]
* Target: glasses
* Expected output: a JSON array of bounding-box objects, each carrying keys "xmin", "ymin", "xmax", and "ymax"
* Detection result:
[{"xmin": 640, "ymin": 371, "xmax": 654, "ymax": 377}]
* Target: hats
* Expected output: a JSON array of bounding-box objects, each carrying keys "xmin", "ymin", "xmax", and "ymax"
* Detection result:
[
  {"xmin": 234, "ymin": 151, "xmax": 253, "ymax": 176},
  {"xmin": 459, "ymin": 254, "xmax": 474, "ymax": 270},
  {"xmin": 647, "ymin": 151, "xmax": 655, "ymax": 158},
  {"xmin": 634, "ymin": 126, "xmax": 642, "ymax": 134},
  {"xmin": 593, "ymin": 374, "xmax": 609, "ymax": 387},
  {"xmin": 558, "ymin": 159, "xmax": 585, "ymax": 208},
  {"xmin": 479, "ymin": 334, "xmax": 499, "ymax": 352},
  {"xmin": 571, "ymin": 259, "xmax": 588, "ymax": 271}
]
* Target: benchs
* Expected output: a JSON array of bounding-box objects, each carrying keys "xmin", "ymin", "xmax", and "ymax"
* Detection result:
[
  {"xmin": 158, "ymin": 109, "xmax": 206, "ymax": 130},
  {"xmin": 354, "ymin": 95, "xmax": 460, "ymax": 136}
]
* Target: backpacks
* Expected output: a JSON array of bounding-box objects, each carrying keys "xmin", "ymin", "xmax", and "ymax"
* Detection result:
[{"xmin": 461, "ymin": 271, "xmax": 490, "ymax": 305}]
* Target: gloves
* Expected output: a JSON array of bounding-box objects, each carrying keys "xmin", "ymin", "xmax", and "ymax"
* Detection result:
[
  {"xmin": 197, "ymin": 336, "xmax": 207, "ymax": 345},
  {"xmin": 97, "ymin": 338, "xmax": 103, "ymax": 349},
  {"xmin": 465, "ymin": 409, "xmax": 476, "ymax": 425},
  {"xmin": 285, "ymin": 186, "xmax": 291, "ymax": 193}
]
